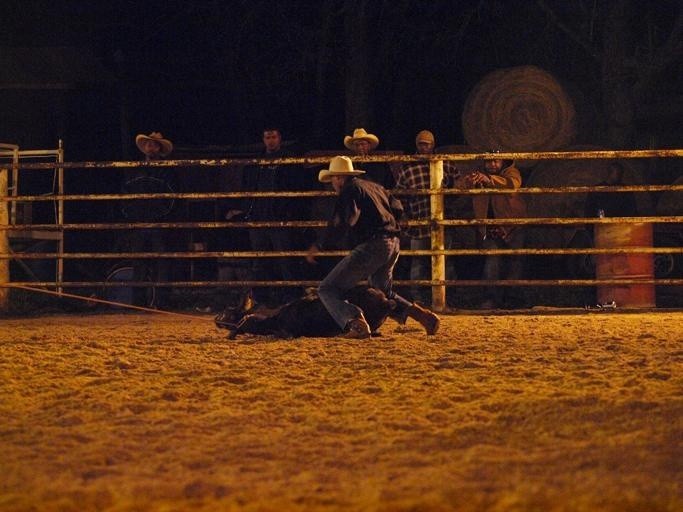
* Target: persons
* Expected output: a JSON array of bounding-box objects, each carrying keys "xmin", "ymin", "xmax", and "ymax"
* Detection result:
[
  {"xmin": 342, "ymin": 127, "xmax": 396, "ymax": 190},
  {"xmin": 126, "ymin": 131, "xmax": 182, "ymax": 313},
  {"xmin": 397, "ymin": 130, "xmax": 468, "ymax": 303},
  {"xmin": 316, "ymin": 154, "xmax": 440, "ymax": 341},
  {"xmin": 452, "ymin": 145, "xmax": 528, "ymax": 307}
]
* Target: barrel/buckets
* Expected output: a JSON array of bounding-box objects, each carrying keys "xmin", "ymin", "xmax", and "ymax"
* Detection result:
[{"xmin": 594, "ymin": 221, "xmax": 656, "ymax": 308}]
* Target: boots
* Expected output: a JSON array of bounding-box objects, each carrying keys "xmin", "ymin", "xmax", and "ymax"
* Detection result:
[
  {"xmin": 341, "ymin": 313, "xmax": 372, "ymax": 339},
  {"xmin": 408, "ymin": 303, "xmax": 440, "ymax": 335}
]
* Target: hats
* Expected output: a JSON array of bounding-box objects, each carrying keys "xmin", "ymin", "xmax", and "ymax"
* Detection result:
[
  {"xmin": 344, "ymin": 128, "xmax": 380, "ymax": 150},
  {"xmin": 136, "ymin": 132, "xmax": 173, "ymax": 157},
  {"xmin": 416, "ymin": 131, "xmax": 435, "ymax": 146},
  {"xmin": 318, "ymin": 155, "xmax": 367, "ymax": 183}
]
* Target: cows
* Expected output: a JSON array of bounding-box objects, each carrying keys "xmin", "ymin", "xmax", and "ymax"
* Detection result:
[{"xmin": 213, "ymin": 283, "xmax": 396, "ymax": 339}]
